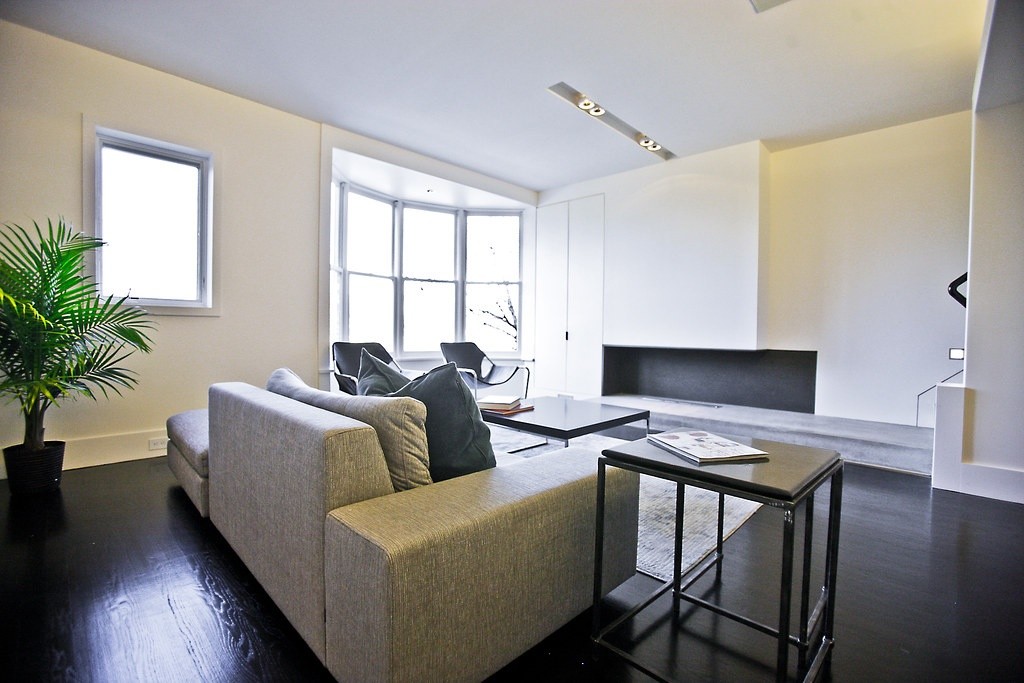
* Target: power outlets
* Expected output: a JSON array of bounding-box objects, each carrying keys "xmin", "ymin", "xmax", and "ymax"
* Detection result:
[{"xmin": 148, "ymin": 437, "xmax": 168, "ymax": 450}]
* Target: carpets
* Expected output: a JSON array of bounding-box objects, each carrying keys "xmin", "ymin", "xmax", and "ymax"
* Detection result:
[{"xmin": 487, "ymin": 426, "xmax": 765, "ymax": 582}]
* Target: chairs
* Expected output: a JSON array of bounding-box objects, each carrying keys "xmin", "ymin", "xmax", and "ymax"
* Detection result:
[
  {"xmin": 438, "ymin": 341, "xmax": 531, "ymax": 399},
  {"xmin": 329, "ymin": 343, "xmax": 429, "ymax": 396}
]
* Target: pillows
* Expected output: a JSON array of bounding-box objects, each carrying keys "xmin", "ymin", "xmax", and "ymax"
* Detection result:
[{"xmin": 356, "ymin": 346, "xmax": 497, "ymax": 481}]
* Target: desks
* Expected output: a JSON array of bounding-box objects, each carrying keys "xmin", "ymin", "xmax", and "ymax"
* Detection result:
[{"xmin": 586, "ymin": 426, "xmax": 845, "ymax": 683}]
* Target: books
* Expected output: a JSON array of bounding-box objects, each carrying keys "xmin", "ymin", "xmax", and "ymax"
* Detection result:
[
  {"xmin": 647, "ymin": 430, "xmax": 770, "ymax": 464},
  {"xmin": 477, "ymin": 394, "xmax": 535, "ymax": 415}
]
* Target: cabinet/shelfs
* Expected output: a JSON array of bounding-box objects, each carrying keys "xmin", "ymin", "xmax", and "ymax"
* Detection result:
[{"xmin": 535, "ymin": 194, "xmax": 625, "ymax": 400}]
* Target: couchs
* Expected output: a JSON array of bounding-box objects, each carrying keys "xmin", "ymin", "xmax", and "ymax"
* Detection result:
[{"xmin": 163, "ymin": 367, "xmax": 642, "ymax": 683}]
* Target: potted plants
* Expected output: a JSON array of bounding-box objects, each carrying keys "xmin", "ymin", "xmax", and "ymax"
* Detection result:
[{"xmin": 0, "ymin": 214, "xmax": 160, "ymax": 501}]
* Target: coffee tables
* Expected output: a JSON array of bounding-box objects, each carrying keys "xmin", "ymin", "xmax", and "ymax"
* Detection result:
[{"xmin": 483, "ymin": 397, "xmax": 651, "ymax": 455}]
